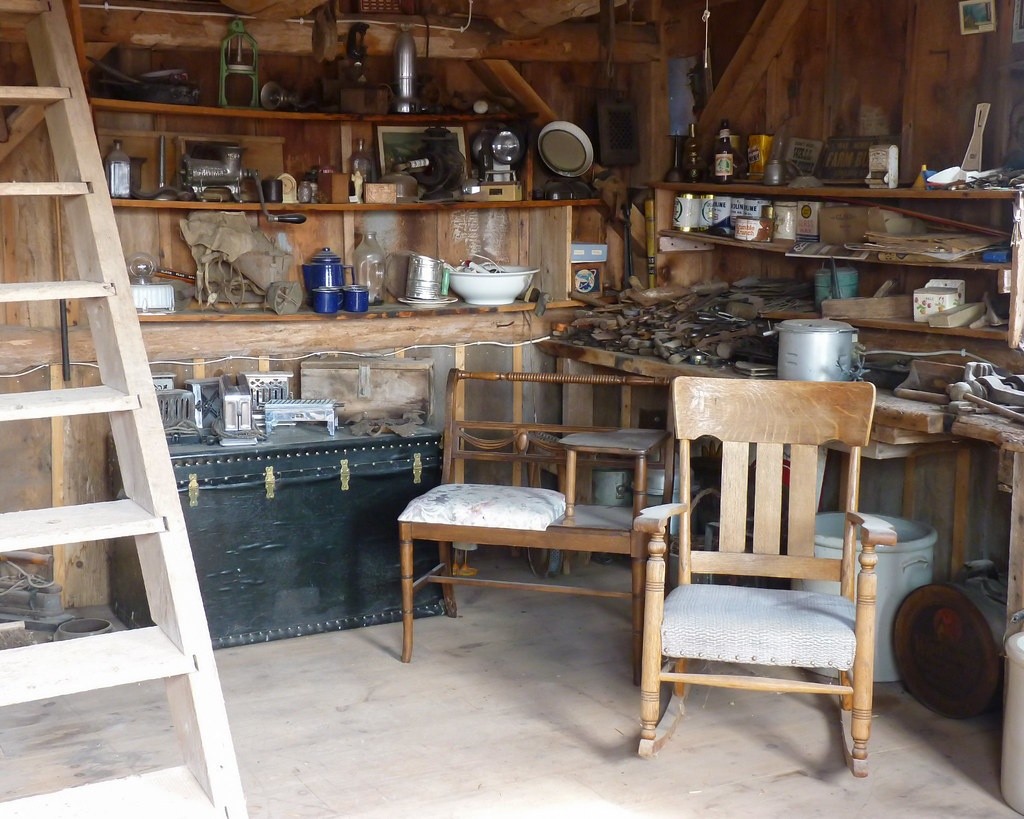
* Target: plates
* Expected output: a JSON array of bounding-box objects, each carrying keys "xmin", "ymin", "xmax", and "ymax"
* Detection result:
[{"xmin": 397, "ymin": 297, "xmax": 458, "ymax": 307}]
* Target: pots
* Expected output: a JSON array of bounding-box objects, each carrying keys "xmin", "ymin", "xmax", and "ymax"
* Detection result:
[
  {"xmin": 774, "ymin": 319, "xmax": 859, "ymax": 382},
  {"xmin": 590, "ymin": 449, "xmax": 696, "ymax": 538}
]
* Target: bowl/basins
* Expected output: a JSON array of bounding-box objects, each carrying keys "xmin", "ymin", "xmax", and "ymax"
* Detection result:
[
  {"xmin": 141, "ymin": 68, "xmax": 187, "ymax": 83},
  {"xmin": 446, "ymin": 265, "xmax": 540, "ymax": 305}
]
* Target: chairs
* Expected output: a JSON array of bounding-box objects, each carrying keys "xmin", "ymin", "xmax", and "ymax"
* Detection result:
[{"xmin": 634, "ymin": 375, "xmax": 897, "ymax": 779}]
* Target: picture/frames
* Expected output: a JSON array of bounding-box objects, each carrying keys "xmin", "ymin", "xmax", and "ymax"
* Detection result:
[{"xmin": 370, "ymin": 121, "xmax": 473, "ymax": 183}]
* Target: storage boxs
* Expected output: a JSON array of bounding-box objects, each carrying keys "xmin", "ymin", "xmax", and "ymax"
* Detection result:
[
  {"xmin": 571, "ymin": 243, "xmax": 609, "ymax": 264},
  {"xmin": 298, "ymin": 354, "xmax": 434, "ymax": 422},
  {"xmin": 819, "ymin": 208, "xmax": 903, "ymax": 244},
  {"xmin": 106, "ymin": 420, "xmax": 448, "ymax": 651}
]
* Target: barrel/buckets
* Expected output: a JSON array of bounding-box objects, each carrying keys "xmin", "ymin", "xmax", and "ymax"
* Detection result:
[
  {"xmin": 815, "ymin": 258, "xmax": 858, "ymax": 311},
  {"xmin": 1000, "ymin": 609, "xmax": 1024, "ymax": 816},
  {"xmin": 791, "ymin": 511, "xmax": 938, "ymax": 682}
]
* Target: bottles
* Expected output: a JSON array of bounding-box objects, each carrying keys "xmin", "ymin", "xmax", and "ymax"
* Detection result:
[
  {"xmin": 104, "ymin": 140, "xmax": 130, "ymax": 199},
  {"xmin": 763, "ymin": 159, "xmax": 786, "ymax": 186},
  {"xmin": 685, "ymin": 123, "xmax": 705, "ymax": 183},
  {"xmin": 353, "ymin": 231, "xmax": 387, "ymax": 306},
  {"xmin": 350, "ymin": 138, "xmax": 371, "ymax": 202},
  {"xmin": 713, "ymin": 119, "xmax": 735, "ymax": 183}
]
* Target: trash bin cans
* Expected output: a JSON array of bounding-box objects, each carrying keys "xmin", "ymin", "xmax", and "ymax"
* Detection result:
[{"xmin": 791, "ymin": 510, "xmax": 940, "ymax": 685}]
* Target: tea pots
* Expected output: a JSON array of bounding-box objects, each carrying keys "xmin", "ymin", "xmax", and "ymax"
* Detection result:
[{"xmin": 302, "ymin": 247, "xmax": 355, "ymax": 306}]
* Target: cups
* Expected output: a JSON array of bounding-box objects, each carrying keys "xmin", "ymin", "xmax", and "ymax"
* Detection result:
[
  {"xmin": 314, "ymin": 285, "xmax": 369, "ymax": 313},
  {"xmin": 261, "ymin": 179, "xmax": 283, "ymax": 203}
]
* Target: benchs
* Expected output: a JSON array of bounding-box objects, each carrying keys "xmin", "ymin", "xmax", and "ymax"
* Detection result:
[{"xmin": 395, "ymin": 367, "xmax": 677, "ymax": 686}]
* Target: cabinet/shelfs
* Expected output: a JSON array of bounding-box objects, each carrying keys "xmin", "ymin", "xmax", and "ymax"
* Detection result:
[
  {"xmin": 91, "ymin": 97, "xmax": 621, "ymax": 322},
  {"xmin": 653, "ymin": 180, "xmax": 1024, "ymax": 349}
]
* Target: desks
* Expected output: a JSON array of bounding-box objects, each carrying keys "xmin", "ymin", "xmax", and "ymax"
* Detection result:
[
  {"xmin": 533, "ymin": 341, "xmax": 970, "ymax": 581},
  {"xmin": 559, "ymin": 427, "xmax": 672, "ymax": 533},
  {"xmin": 950, "ymin": 413, "xmax": 1023, "ymax": 641}
]
